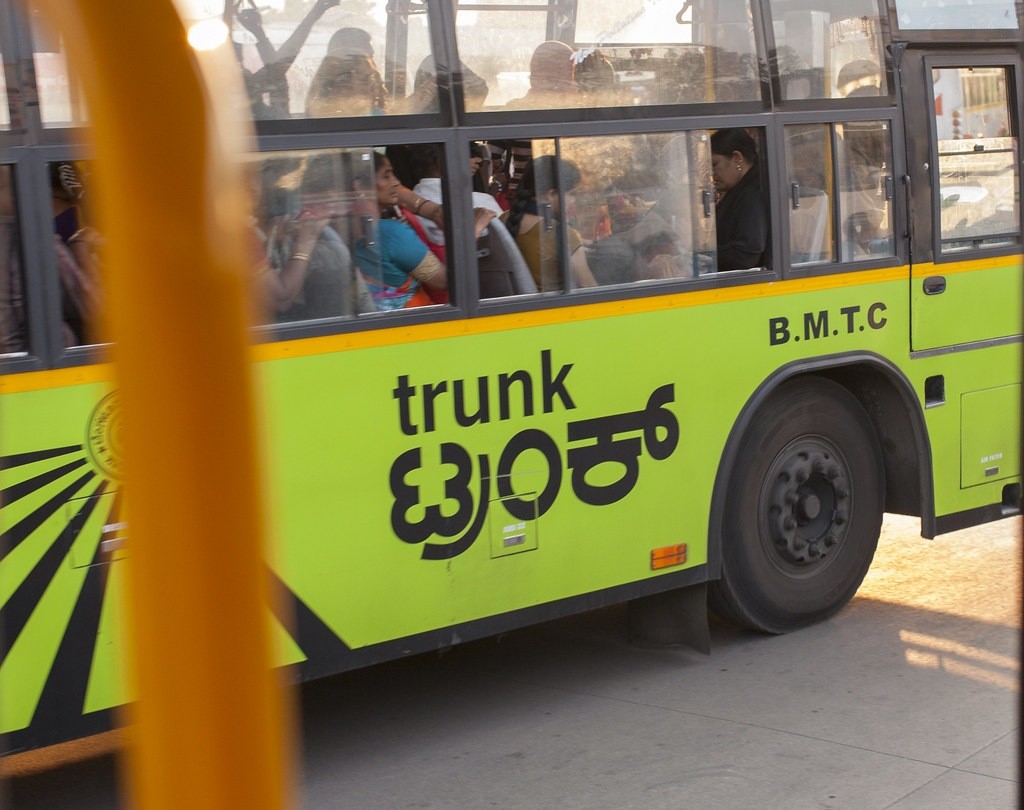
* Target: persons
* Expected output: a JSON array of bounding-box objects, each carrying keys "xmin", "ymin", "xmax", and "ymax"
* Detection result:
[
  {"xmin": 242, "ymin": 162, "xmax": 331, "ymax": 316},
  {"xmin": 498, "ymin": 154, "xmax": 599, "ymax": 293},
  {"xmin": 235, "ymin": 0, "xmax": 438, "ymax": 121},
  {"xmin": 640, "ymin": 127, "xmax": 768, "ymax": 280},
  {"xmin": 0, "ymin": 160, "xmax": 93, "ymax": 355},
  {"xmin": 299, "ymin": 150, "xmax": 498, "ymax": 311},
  {"xmin": 411, "ymin": 141, "xmax": 504, "ymax": 246},
  {"xmin": 501, "ymin": 39, "xmax": 614, "ymax": 111}
]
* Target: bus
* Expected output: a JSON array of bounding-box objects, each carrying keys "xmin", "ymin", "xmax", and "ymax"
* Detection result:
[{"xmin": 0, "ymin": 0, "xmax": 1024, "ymax": 791}]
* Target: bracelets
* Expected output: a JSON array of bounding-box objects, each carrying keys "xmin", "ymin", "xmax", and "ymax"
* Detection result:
[
  {"xmin": 415, "ymin": 200, "xmax": 430, "ymax": 214},
  {"xmin": 414, "ymin": 197, "xmax": 421, "ymax": 210},
  {"xmin": 288, "ymin": 253, "xmax": 310, "ymax": 262}
]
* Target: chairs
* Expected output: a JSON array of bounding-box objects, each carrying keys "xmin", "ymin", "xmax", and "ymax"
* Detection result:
[
  {"xmin": 480, "ymin": 217, "xmax": 537, "ymax": 299},
  {"xmin": 789, "ymin": 186, "xmax": 828, "ymax": 262},
  {"xmin": 279, "ymin": 221, "xmax": 354, "ymax": 318}
]
